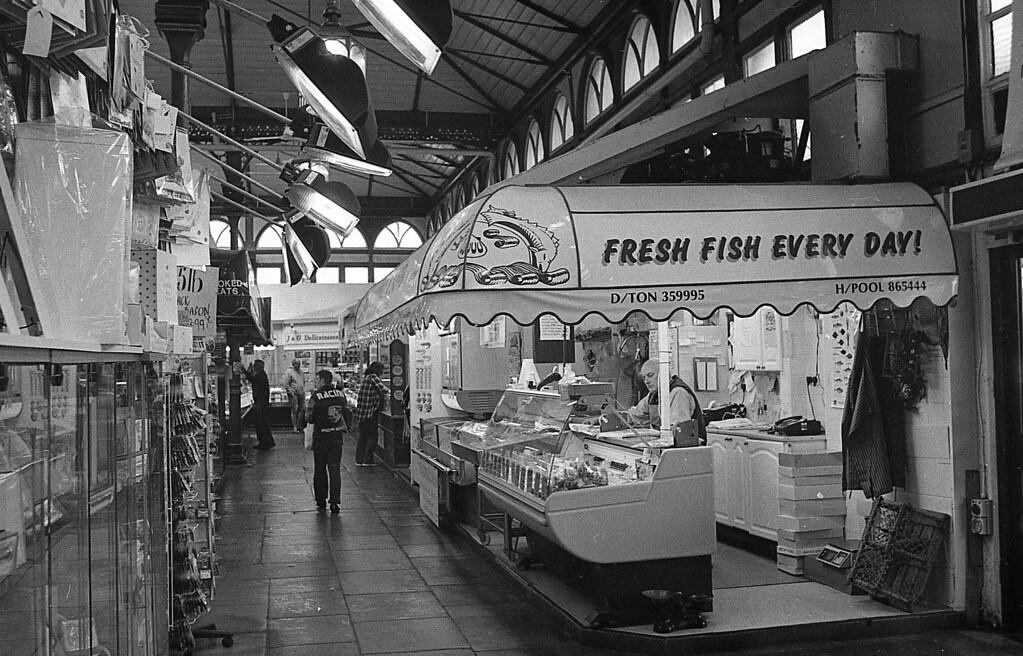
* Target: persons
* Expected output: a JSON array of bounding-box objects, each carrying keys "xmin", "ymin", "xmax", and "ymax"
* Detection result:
[
  {"xmin": 582, "ymin": 358, "xmax": 707, "ymax": 447},
  {"xmin": 284, "ymin": 358, "xmax": 305, "ymax": 434},
  {"xmin": 355, "ymin": 361, "xmax": 391, "ymax": 466},
  {"xmin": 241, "ymin": 359, "xmax": 276, "ymax": 450},
  {"xmin": 304, "ymin": 370, "xmax": 348, "ymax": 512}
]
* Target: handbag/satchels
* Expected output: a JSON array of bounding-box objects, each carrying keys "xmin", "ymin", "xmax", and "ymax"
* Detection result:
[{"xmin": 303, "ymin": 422, "xmax": 314, "ymax": 451}]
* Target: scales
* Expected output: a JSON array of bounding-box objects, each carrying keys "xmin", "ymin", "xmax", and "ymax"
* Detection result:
[{"xmin": 816, "ymin": 539, "xmax": 872, "ymax": 568}]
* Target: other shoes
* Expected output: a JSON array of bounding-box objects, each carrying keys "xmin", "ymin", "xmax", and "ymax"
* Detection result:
[
  {"xmin": 293, "ymin": 426, "xmax": 303, "ymax": 433},
  {"xmin": 317, "ymin": 500, "xmax": 327, "ymax": 508},
  {"xmin": 330, "ymin": 503, "xmax": 341, "ymax": 514},
  {"xmin": 356, "ymin": 461, "xmax": 378, "ymax": 467},
  {"xmin": 253, "ymin": 443, "xmax": 276, "ymax": 450}
]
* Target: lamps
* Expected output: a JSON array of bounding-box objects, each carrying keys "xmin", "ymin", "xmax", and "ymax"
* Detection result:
[{"xmin": 145, "ymin": 0, "xmax": 455, "ymax": 287}]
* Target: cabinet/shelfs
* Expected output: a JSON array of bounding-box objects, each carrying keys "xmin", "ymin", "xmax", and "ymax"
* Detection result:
[
  {"xmin": 704, "ymin": 427, "xmax": 827, "ymax": 560},
  {"xmin": 732, "ymin": 313, "xmax": 784, "ymax": 371}
]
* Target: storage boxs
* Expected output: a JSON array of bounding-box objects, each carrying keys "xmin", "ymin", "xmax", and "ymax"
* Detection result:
[{"xmin": 776, "ymin": 453, "xmax": 869, "ymax": 597}]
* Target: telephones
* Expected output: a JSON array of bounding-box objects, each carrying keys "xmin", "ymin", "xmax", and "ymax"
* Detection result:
[{"xmin": 767, "ymin": 415, "xmax": 821, "ymax": 435}]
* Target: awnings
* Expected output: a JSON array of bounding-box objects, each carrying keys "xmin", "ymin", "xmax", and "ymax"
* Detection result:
[{"xmin": 354, "ymin": 184, "xmax": 960, "ymax": 345}]
